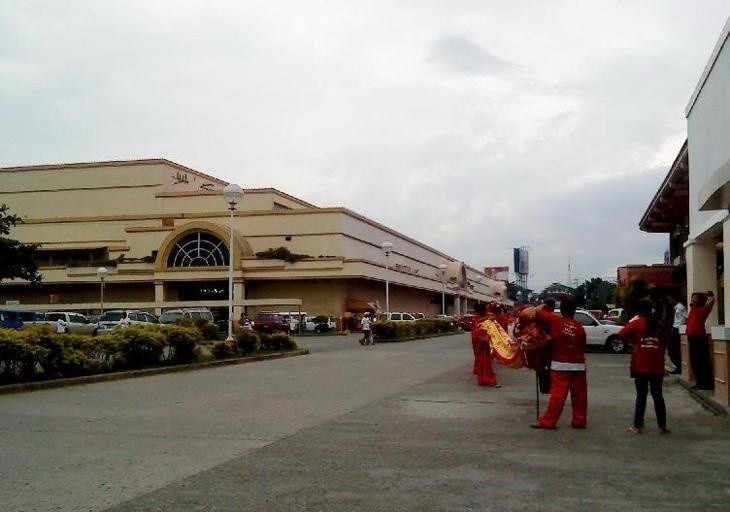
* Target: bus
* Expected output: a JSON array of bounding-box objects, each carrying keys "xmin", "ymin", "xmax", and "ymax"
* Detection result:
[{"xmin": 0, "ymin": 310, "xmax": 45, "ymax": 331}]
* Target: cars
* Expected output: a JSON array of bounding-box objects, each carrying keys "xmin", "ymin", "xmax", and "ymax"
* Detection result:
[
  {"xmin": 96, "ymin": 310, "xmax": 161, "ymax": 336},
  {"xmin": 379, "ymin": 311, "xmax": 473, "ymax": 331},
  {"xmin": 254, "ymin": 310, "xmax": 336, "ymax": 334},
  {"xmin": 552, "ymin": 307, "xmax": 626, "ymax": 354}
]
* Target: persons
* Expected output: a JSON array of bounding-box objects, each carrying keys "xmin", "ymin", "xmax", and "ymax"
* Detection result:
[
  {"xmin": 617, "ymin": 299, "xmax": 673, "ymax": 435},
  {"xmin": 238, "ymin": 313, "xmax": 254, "ymax": 329},
  {"xmin": 358, "ymin": 311, "xmax": 374, "ymax": 345},
  {"xmin": 663, "ymin": 292, "xmax": 687, "ymax": 375},
  {"xmin": 471, "ymin": 296, "xmax": 557, "ymax": 394},
  {"xmin": 685, "ymin": 289, "xmax": 716, "ymax": 390},
  {"xmin": 56, "ymin": 314, "xmax": 67, "ymax": 334},
  {"xmin": 120, "ymin": 312, "xmax": 132, "ymax": 328},
  {"xmin": 529, "ymin": 297, "xmax": 589, "ymax": 429}
]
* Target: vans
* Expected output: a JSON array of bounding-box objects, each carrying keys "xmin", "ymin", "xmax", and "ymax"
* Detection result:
[{"xmin": 157, "ymin": 308, "xmax": 215, "ymax": 334}]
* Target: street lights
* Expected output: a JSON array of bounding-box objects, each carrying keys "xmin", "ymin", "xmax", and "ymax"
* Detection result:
[
  {"xmin": 476, "ymin": 276, "xmax": 483, "ymax": 304},
  {"xmin": 222, "ymin": 183, "xmax": 244, "ymax": 341},
  {"xmin": 381, "ymin": 241, "xmax": 393, "ymax": 320},
  {"xmin": 439, "ymin": 263, "xmax": 448, "ymax": 317},
  {"xmin": 97, "ymin": 266, "xmax": 108, "ymax": 315}
]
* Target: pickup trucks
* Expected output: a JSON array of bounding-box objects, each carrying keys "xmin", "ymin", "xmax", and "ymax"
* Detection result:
[{"xmin": 21, "ymin": 311, "xmax": 100, "ymax": 337}]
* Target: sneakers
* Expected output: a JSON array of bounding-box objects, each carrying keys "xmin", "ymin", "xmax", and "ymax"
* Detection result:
[{"xmin": 529, "ymin": 421, "xmax": 556, "ymax": 429}]
